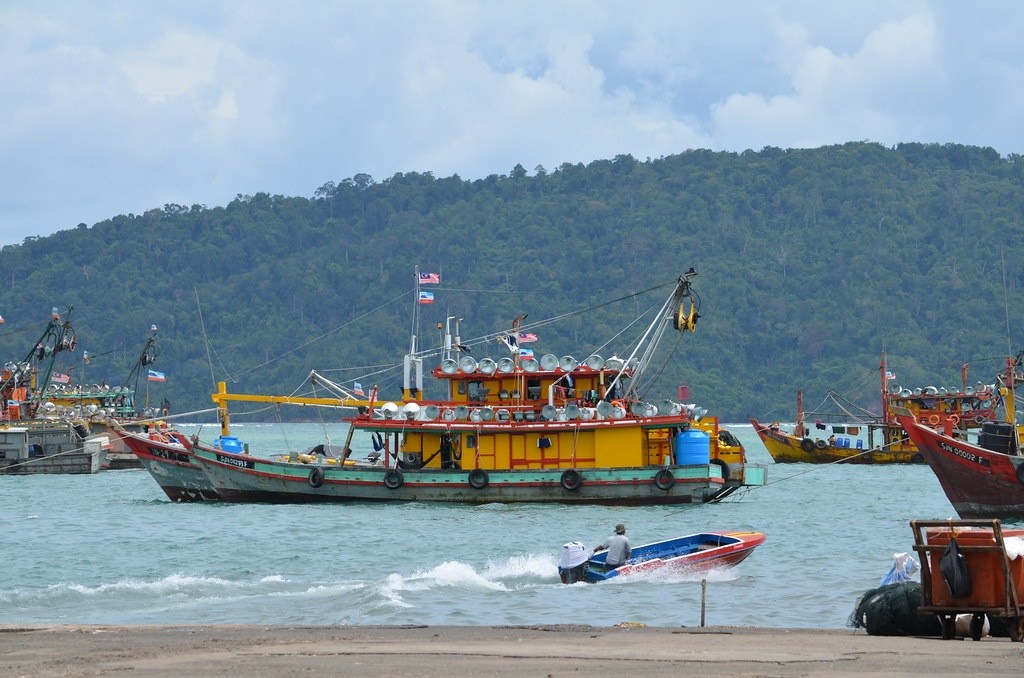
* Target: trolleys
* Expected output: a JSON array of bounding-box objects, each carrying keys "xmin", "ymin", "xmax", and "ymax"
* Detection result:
[{"xmin": 908, "ymin": 516, "xmax": 1024, "ymax": 642}]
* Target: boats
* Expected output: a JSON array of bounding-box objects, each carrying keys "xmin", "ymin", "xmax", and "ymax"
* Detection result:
[
  {"xmin": 748, "ymin": 348, "xmax": 1023, "ymax": 463},
  {"xmin": 108, "ymin": 267, "xmax": 767, "ymax": 505},
  {"xmin": 558, "ymin": 531, "xmax": 766, "ymax": 585},
  {"xmin": 0, "ymin": 305, "xmax": 169, "ymax": 474},
  {"xmin": 888, "ymin": 387, "xmax": 1023, "ymax": 524}
]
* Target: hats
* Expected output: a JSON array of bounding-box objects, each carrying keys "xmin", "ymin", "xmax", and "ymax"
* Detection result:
[{"xmin": 613, "ymin": 524, "xmax": 628, "ymax": 532}]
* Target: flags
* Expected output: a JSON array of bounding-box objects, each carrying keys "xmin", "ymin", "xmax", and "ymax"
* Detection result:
[
  {"xmin": 148, "ymin": 370, "xmax": 165, "ymax": 382},
  {"xmin": 518, "ymin": 333, "xmax": 537, "ymax": 343},
  {"xmin": 52, "ymin": 372, "xmax": 69, "ymax": 383},
  {"xmin": 354, "ymin": 382, "xmax": 364, "ymax": 396},
  {"xmin": 419, "ymin": 292, "xmax": 434, "ymax": 303},
  {"xmin": 419, "ymin": 272, "xmax": 439, "ymax": 284},
  {"xmin": 370, "ymin": 390, "xmax": 377, "ymax": 402},
  {"xmin": 84, "ymin": 351, "xmax": 89, "ymax": 365},
  {"xmin": 886, "ymin": 371, "xmax": 895, "ymax": 380}
]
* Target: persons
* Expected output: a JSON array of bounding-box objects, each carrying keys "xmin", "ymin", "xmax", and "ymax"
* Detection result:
[
  {"xmin": 828, "ymin": 435, "xmax": 836, "ymax": 446},
  {"xmin": 358, "ymin": 406, "xmax": 374, "ymax": 417},
  {"xmin": 594, "ymin": 524, "xmax": 631, "ymax": 570}
]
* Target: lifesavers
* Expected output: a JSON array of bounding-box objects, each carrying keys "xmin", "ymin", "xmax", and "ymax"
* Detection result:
[
  {"xmin": 162, "ymin": 407, "xmax": 169, "ymax": 419},
  {"xmin": 928, "ymin": 414, "xmax": 940, "ymax": 427},
  {"xmin": 801, "ymin": 437, "xmax": 827, "ymax": 453},
  {"xmin": 468, "ymin": 467, "xmax": 489, "ymax": 490},
  {"xmin": 950, "ymin": 413, "xmax": 960, "ymax": 424},
  {"xmin": 561, "ymin": 468, "xmax": 583, "ymax": 490},
  {"xmin": 860, "ymin": 448, "xmax": 870, "ymax": 460},
  {"xmin": 975, "ymin": 415, "xmax": 984, "ymax": 424},
  {"xmin": 610, "ymin": 400, "xmax": 625, "ymax": 410},
  {"xmin": 911, "ymin": 451, "xmax": 926, "ymax": 465},
  {"xmin": 308, "ymin": 465, "xmax": 325, "ymax": 489},
  {"xmin": 653, "ymin": 468, "xmax": 677, "ymax": 490},
  {"xmin": 384, "ymin": 469, "xmax": 405, "ymax": 490}
]
